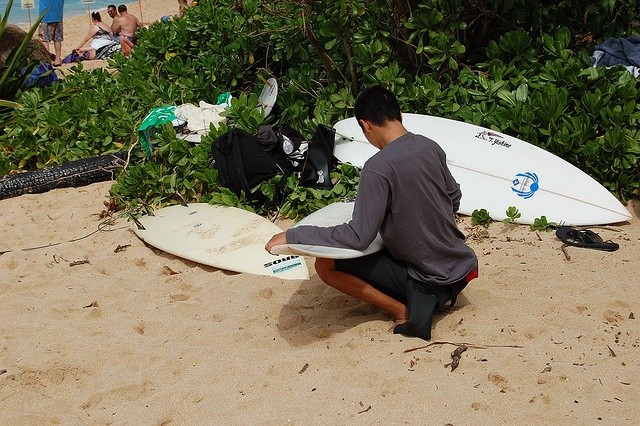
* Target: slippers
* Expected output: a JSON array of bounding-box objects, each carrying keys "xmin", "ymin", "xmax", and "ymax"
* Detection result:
[
  {"xmin": 562, "ymin": 231, "xmax": 620, "ymax": 250},
  {"xmin": 556, "ymin": 227, "xmax": 583, "ymax": 239}
]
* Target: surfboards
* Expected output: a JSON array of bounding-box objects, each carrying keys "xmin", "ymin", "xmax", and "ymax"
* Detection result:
[
  {"xmin": 270, "ymin": 202, "xmax": 385, "ymax": 259},
  {"xmin": 333, "ymin": 112, "xmax": 633, "ymax": 226},
  {"xmin": 126, "ymin": 188, "xmax": 311, "ymax": 280}
]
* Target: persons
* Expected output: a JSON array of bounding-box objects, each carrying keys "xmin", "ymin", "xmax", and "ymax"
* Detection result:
[
  {"xmin": 36, "ymin": 0, "xmax": 64, "ymax": 67},
  {"xmin": 265, "ymin": 85, "xmax": 479, "ymax": 335},
  {"xmin": 113, "ymin": 5, "xmax": 145, "ymax": 42},
  {"xmin": 178, "ymin": 1, "xmax": 197, "ymax": 17},
  {"xmin": 106, "ymin": 6, "xmax": 121, "ymax": 22},
  {"xmin": 73, "ymin": 12, "xmax": 114, "ymax": 60}
]
